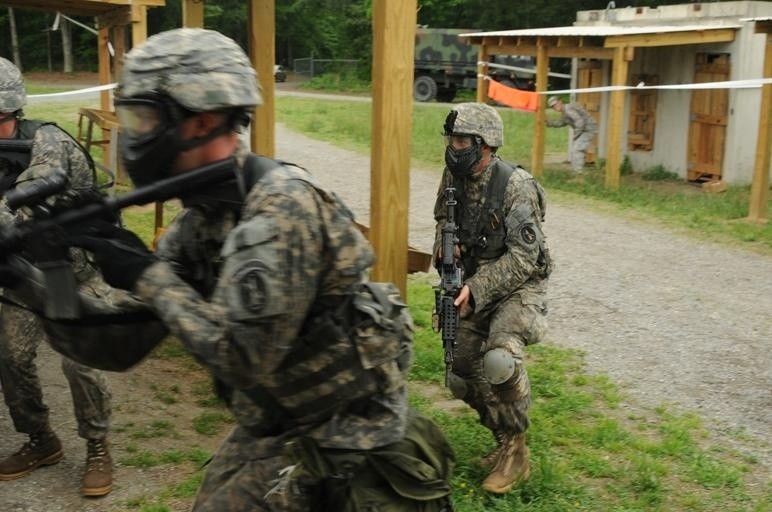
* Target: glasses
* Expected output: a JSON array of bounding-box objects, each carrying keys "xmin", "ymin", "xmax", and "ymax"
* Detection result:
[
  {"xmin": 112, "ymin": 98, "xmax": 171, "ymax": 137},
  {"xmin": 443, "ymin": 135, "xmax": 475, "ymax": 150}
]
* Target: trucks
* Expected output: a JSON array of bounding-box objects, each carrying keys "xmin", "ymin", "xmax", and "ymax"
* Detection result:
[{"xmin": 414, "ymin": 26, "xmax": 570, "ymax": 103}]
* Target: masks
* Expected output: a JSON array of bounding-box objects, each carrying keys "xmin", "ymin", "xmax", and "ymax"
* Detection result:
[
  {"xmin": 114, "ymin": 128, "xmax": 180, "ymax": 194},
  {"xmin": 444, "ymin": 144, "xmax": 481, "ymax": 177}
]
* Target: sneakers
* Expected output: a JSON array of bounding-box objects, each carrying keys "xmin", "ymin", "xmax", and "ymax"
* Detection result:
[{"xmin": 566, "ymin": 173, "xmax": 584, "ymax": 185}]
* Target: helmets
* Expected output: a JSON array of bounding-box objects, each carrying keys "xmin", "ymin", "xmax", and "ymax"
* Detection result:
[
  {"xmin": 0, "ymin": 56, "xmax": 27, "ymax": 114},
  {"xmin": 443, "ymin": 102, "xmax": 504, "ymax": 149},
  {"xmin": 111, "ymin": 23, "xmax": 266, "ymax": 111}
]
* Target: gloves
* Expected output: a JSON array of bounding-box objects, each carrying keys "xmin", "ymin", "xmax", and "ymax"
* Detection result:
[
  {"xmin": 0, "ymin": 252, "xmax": 33, "ymax": 290},
  {"xmin": 65, "ymin": 227, "xmax": 162, "ymax": 293}
]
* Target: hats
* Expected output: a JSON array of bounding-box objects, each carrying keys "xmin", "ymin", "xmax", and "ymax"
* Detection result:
[{"xmin": 547, "ymin": 95, "xmax": 559, "ymax": 108}]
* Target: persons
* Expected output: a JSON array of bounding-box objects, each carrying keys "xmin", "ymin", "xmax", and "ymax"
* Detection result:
[
  {"xmin": 0, "ymin": 58, "xmax": 122, "ymax": 496},
  {"xmin": 430, "ymin": 102, "xmax": 555, "ymax": 495},
  {"xmin": 0, "ymin": 28, "xmax": 451, "ymax": 512},
  {"xmin": 543, "ymin": 95, "xmax": 599, "ymax": 185}
]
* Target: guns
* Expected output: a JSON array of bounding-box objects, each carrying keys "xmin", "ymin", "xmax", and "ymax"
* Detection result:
[
  {"xmin": 1, "ymin": 156, "xmax": 243, "ymax": 319},
  {"xmin": 431, "ymin": 187, "xmax": 462, "ymax": 372},
  {"xmin": 0, "ymin": 139, "xmax": 33, "ymax": 186}
]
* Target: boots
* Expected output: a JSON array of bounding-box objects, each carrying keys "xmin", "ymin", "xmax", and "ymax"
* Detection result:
[
  {"xmin": 0, "ymin": 416, "xmax": 68, "ymax": 481},
  {"xmin": 471, "ymin": 429, "xmax": 535, "ymax": 496},
  {"xmin": 78, "ymin": 433, "xmax": 115, "ymax": 496}
]
